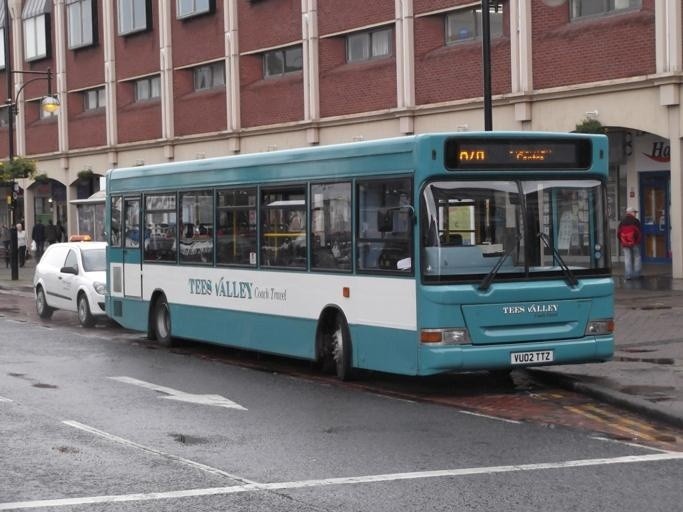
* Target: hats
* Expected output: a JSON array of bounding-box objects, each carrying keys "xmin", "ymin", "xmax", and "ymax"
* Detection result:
[{"xmin": 625, "ymin": 207, "xmax": 640, "ymax": 213}]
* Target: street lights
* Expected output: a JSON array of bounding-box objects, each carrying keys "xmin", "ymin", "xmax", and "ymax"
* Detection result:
[{"xmin": 4, "ymin": 0, "xmax": 59, "ymax": 283}]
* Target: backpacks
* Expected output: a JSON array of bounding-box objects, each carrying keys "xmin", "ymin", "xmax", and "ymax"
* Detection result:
[{"xmin": 618, "ymin": 217, "xmax": 642, "ymax": 247}]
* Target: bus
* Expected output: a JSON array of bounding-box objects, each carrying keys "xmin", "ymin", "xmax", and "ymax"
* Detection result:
[{"xmin": 101, "ymin": 130, "xmax": 618, "ymax": 386}]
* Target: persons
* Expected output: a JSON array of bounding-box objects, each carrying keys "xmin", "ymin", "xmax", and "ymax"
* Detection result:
[
  {"xmin": 617, "ymin": 207, "xmax": 646, "ymax": 281},
  {"xmin": 0, "ymin": 218, "xmax": 68, "ymax": 268}
]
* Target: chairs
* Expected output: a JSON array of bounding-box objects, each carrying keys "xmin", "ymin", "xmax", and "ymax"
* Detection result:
[
  {"xmin": 439, "ymin": 234, "xmax": 462, "ymax": 246},
  {"xmin": 314, "ymin": 248, "xmax": 351, "ymax": 269}
]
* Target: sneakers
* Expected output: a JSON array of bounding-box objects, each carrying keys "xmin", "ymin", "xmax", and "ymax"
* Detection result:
[{"xmin": 623, "ymin": 273, "xmax": 646, "ymax": 285}]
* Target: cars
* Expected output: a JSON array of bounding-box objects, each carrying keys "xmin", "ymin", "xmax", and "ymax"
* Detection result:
[{"xmin": 33, "ymin": 234, "xmax": 109, "ymax": 328}]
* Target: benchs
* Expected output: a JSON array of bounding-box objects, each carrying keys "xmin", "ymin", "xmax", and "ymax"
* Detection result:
[
  {"xmin": 612, "ymin": 270, "xmax": 671, "ymax": 290},
  {"xmin": 0, "ymin": 248, "xmax": 11, "ymax": 268}
]
